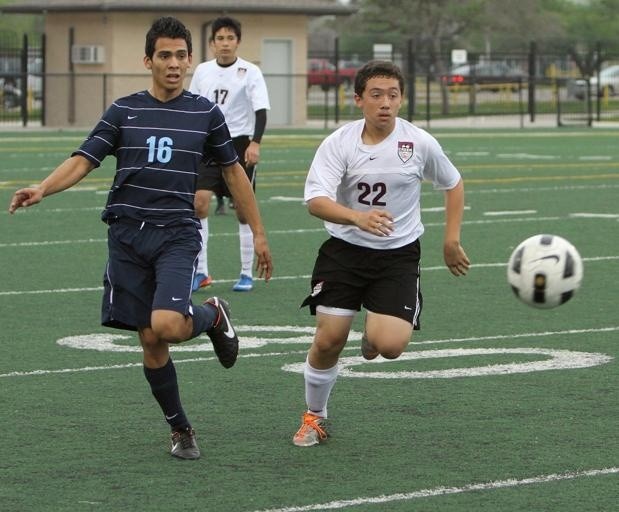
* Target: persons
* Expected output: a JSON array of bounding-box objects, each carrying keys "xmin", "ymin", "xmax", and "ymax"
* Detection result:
[
  {"xmin": 8, "ymin": 14, "xmax": 275, "ymax": 461},
  {"xmin": 292, "ymin": 57, "xmax": 472, "ymax": 449},
  {"xmin": 185, "ymin": 15, "xmax": 272, "ymax": 293},
  {"xmin": 207, "ymin": 35, "xmax": 237, "ymax": 215}
]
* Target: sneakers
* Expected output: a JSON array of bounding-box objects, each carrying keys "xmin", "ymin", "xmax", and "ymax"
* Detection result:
[
  {"xmin": 202, "ymin": 296, "xmax": 238, "ymax": 369},
  {"xmin": 361, "ymin": 312, "xmax": 380, "ymax": 360},
  {"xmin": 232, "ymin": 274, "xmax": 253, "ymax": 292},
  {"xmin": 170, "ymin": 424, "xmax": 200, "ymax": 460},
  {"xmin": 293, "ymin": 412, "xmax": 332, "ymax": 448},
  {"xmin": 192, "ymin": 272, "xmax": 213, "ymax": 292}
]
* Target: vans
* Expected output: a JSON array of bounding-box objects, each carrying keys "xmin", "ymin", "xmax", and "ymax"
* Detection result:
[{"xmin": 23, "ymin": 56, "xmax": 44, "ymax": 100}]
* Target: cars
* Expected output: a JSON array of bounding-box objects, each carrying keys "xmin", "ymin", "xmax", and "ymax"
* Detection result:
[
  {"xmin": 307, "ymin": 59, "xmax": 355, "ymax": 91},
  {"xmin": 0, "ymin": 76, "xmax": 24, "ymax": 112},
  {"xmin": 440, "ymin": 61, "xmax": 530, "ymax": 92},
  {"xmin": 575, "ymin": 65, "xmax": 619, "ymax": 99}
]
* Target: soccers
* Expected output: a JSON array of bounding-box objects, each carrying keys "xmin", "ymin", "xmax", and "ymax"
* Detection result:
[{"xmin": 508, "ymin": 233, "xmax": 582, "ymax": 309}]
